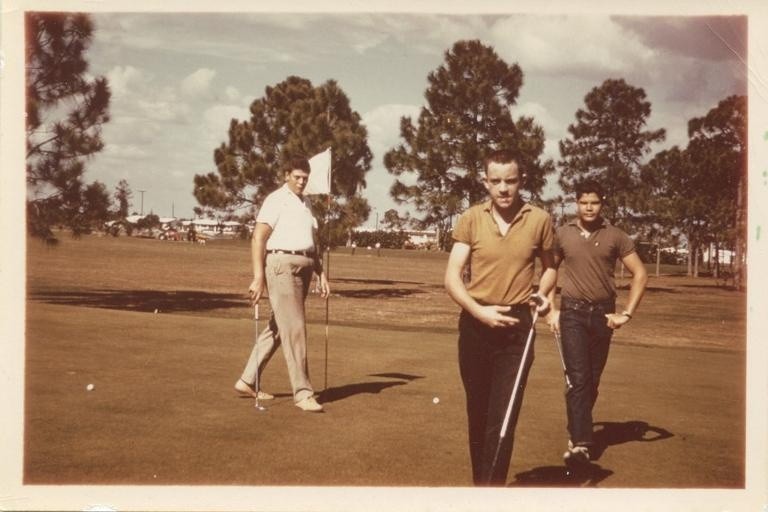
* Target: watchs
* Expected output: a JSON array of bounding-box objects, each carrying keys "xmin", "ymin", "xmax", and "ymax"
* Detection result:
[{"xmin": 621, "ymin": 311, "xmax": 632, "ymax": 319}]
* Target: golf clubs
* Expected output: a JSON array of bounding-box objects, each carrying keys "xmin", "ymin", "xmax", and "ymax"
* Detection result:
[
  {"xmin": 553, "ymin": 329, "xmax": 574, "ymax": 389},
  {"xmin": 254, "ymin": 303, "xmax": 266, "ymax": 412},
  {"xmin": 487, "ymin": 293, "xmax": 544, "ymax": 483}
]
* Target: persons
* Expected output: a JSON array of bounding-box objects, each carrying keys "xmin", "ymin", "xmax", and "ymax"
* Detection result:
[
  {"xmin": 444, "ymin": 150, "xmax": 558, "ymax": 488},
  {"xmin": 353, "ymin": 237, "xmax": 358, "ymax": 255},
  {"xmin": 235, "ymin": 158, "xmax": 330, "ymax": 411},
  {"xmin": 375, "ymin": 240, "xmax": 381, "ymax": 257},
  {"xmin": 546, "ymin": 181, "xmax": 648, "ymax": 468},
  {"xmin": 187, "ymin": 219, "xmax": 196, "ymax": 241}
]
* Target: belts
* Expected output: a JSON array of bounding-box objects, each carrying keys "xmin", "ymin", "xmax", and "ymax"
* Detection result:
[
  {"xmin": 266, "ymin": 249, "xmax": 315, "ymax": 259},
  {"xmin": 560, "ymin": 301, "xmax": 615, "ymax": 312}
]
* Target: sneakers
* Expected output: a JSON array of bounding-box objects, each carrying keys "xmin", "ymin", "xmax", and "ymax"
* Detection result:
[
  {"xmin": 234, "ymin": 378, "xmax": 274, "ymax": 400},
  {"xmin": 559, "ymin": 440, "xmax": 595, "ymax": 488},
  {"xmin": 293, "ymin": 396, "xmax": 323, "ymax": 412}
]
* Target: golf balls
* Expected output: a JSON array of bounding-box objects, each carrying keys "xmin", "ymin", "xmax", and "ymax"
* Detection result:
[
  {"xmin": 86, "ymin": 383, "xmax": 96, "ymax": 392},
  {"xmin": 432, "ymin": 397, "xmax": 440, "ymax": 405}
]
